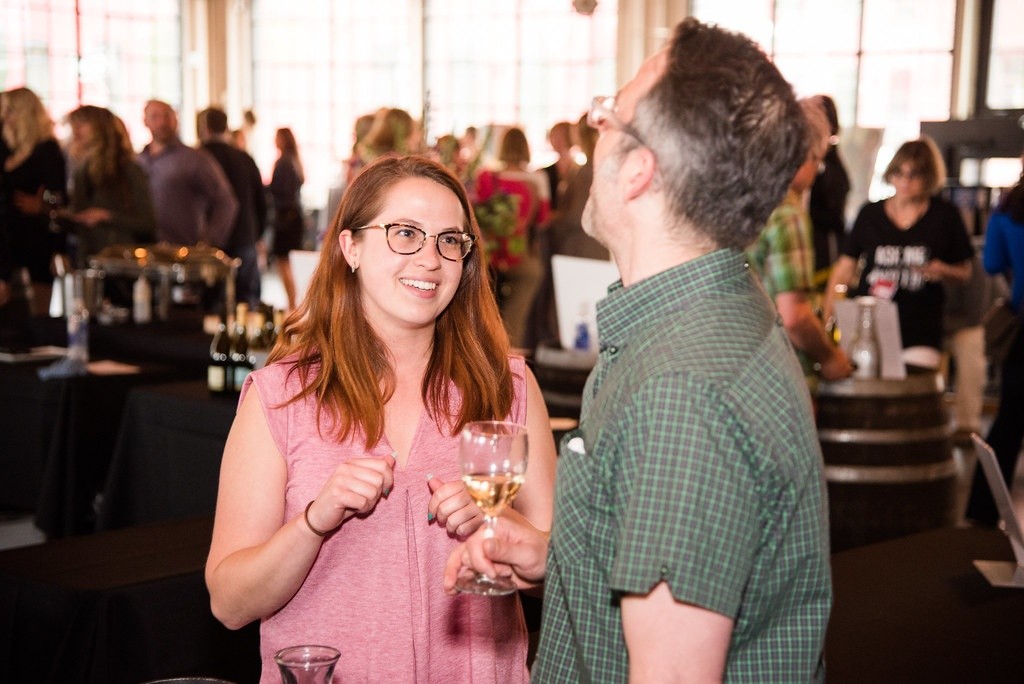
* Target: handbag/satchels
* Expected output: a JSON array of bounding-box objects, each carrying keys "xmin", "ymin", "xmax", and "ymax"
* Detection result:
[
  {"xmin": 931, "ymin": 195, "xmax": 992, "ymax": 338},
  {"xmin": 474, "ymin": 169, "xmax": 521, "ymax": 240}
]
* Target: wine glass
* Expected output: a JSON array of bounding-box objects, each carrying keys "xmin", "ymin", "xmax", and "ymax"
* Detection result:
[{"xmin": 455, "ymin": 420, "xmax": 529, "ymax": 595}]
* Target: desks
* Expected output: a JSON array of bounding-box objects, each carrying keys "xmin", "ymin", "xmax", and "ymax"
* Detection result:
[
  {"xmin": 815, "ymin": 526, "xmax": 1024, "ymax": 684},
  {"xmin": 0, "ymin": 514, "xmax": 259, "ymax": 684}
]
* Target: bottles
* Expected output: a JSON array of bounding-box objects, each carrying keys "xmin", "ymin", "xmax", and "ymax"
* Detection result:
[
  {"xmin": 253, "ymin": 305, "xmax": 278, "ymax": 370},
  {"xmin": 133, "ymin": 275, "xmax": 151, "ymax": 323},
  {"xmin": 851, "ymin": 297, "xmax": 879, "ymax": 380},
  {"xmin": 275, "ymin": 644, "xmax": 341, "ymax": 684},
  {"xmin": 207, "ymin": 307, "xmax": 231, "ymax": 396},
  {"xmin": 232, "ymin": 303, "xmax": 254, "ymax": 395}
]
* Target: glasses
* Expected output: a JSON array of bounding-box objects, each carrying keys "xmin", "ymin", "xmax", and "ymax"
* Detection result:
[
  {"xmin": 813, "ymin": 149, "xmax": 826, "ymax": 175},
  {"xmin": 587, "ymin": 94, "xmax": 647, "ymax": 150},
  {"xmin": 890, "ymin": 167, "xmax": 922, "ymax": 182},
  {"xmin": 355, "ymin": 222, "xmax": 478, "ymax": 262}
]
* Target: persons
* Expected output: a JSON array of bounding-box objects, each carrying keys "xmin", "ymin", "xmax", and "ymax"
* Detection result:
[
  {"xmin": 528, "ymin": 18, "xmax": 831, "ymax": 684},
  {"xmin": 340, "ymin": 107, "xmax": 611, "ymax": 351},
  {"xmin": 1, "ymin": 86, "xmax": 308, "ymax": 315},
  {"xmin": 747, "ymin": 97, "xmax": 1024, "ymax": 525},
  {"xmin": 206, "ymin": 155, "xmax": 555, "ymax": 684}
]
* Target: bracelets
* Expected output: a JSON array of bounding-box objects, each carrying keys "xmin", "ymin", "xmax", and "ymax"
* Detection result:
[{"xmin": 304, "ymin": 500, "xmax": 328, "ymax": 537}]
